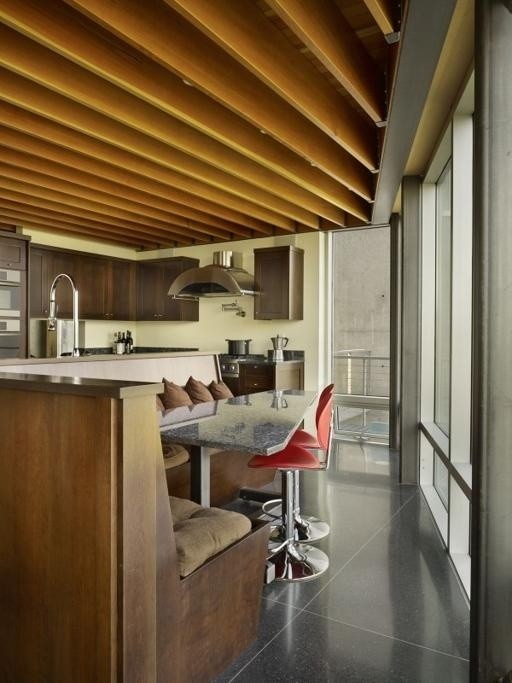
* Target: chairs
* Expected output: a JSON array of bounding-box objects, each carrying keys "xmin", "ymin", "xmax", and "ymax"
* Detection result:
[
  {"xmin": 246, "ymin": 393, "xmax": 334, "ymax": 582},
  {"xmin": 257, "ymin": 382, "xmax": 336, "ymax": 542}
]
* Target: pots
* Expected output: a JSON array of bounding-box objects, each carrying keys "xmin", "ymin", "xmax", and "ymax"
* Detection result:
[{"xmin": 224, "ymin": 338, "xmax": 252, "ymax": 356}]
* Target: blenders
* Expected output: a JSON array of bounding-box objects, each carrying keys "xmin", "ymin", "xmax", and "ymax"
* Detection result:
[{"xmin": 270, "ymin": 333, "xmax": 288, "ymax": 359}]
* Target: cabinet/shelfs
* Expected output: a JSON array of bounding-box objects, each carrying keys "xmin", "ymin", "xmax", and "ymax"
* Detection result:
[
  {"xmin": 137, "ymin": 255, "xmax": 200, "ymax": 322},
  {"xmin": 240, "ymin": 359, "xmax": 306, "ymax": 430},
  {"xmin": 1, "ymin": 231, "xmax": 32, "ymax": 359},
  {"xmin": 75, "ymin": 252, "xmax": 138, "ymax": 321},
  {"xmin": 251, "ymin": 244, "xmax": 305, "ymax": 321},
  {"xmin": 27, "ymin": 242, "xmax": 73, "ymax": 318}
]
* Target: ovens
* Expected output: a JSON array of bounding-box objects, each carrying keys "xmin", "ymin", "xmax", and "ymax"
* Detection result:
[{"xmin": 219, "ymin": 359, "xmax": 246, "ymax": 378}]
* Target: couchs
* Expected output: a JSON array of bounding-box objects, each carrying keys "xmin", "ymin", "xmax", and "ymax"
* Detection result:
[{"xmin": 0, "ymin": 355, "xmax": 274, "ymax": 681}]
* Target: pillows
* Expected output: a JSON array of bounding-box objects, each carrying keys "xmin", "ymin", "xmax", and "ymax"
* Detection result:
[
  {"xmin": 185, "ymin": 373, "xmax": 214, "ymax": 404},
  {"xmin": 208, "ymin": 377, "xmax": 234, "ymax": 401},
  {"xmin": 161, "ymin": 376, "xmax": 192, "ymax": 408}
]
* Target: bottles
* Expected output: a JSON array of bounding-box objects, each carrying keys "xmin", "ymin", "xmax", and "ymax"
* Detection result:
[{"xmin": 112, "ymin": 330, "xmax": 133, "ymax": 354}]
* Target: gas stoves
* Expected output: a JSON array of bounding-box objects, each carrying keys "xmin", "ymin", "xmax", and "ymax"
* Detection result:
[{"xmin": 218, "ymin": 353, "xmax": 264, "ymax": 359}]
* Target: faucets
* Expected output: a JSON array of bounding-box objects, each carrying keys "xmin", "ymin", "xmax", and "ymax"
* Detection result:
[{"xmin": 46, "ymin": 272, "xmax": 80, "ymax": 357}]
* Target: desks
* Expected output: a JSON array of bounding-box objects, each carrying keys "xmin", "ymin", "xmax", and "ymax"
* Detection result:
[{"xmin": 158, "ymin": 387, "xmax": 319, "ymax": 585}]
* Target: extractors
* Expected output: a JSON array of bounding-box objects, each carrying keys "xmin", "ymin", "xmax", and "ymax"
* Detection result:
[{"xmin": 167, "ymin": 250, "xmax": 254, "ymax": 297}]
